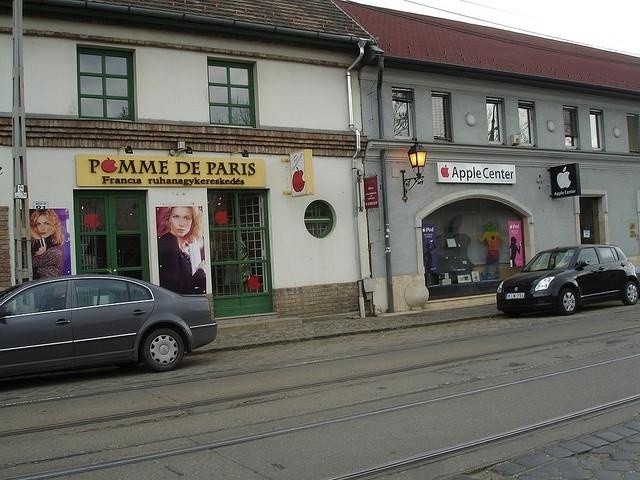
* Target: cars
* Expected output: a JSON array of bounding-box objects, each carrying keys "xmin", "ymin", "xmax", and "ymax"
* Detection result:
[
  {"xmin": 495, "ymin": 245, "xmax": 638, "ymax": 313},
  {"xmin": 2, "ymin": 276, "xmax": 217, "ymax": 381}
]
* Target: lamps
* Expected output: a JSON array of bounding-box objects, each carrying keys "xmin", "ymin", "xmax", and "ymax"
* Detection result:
[{"xmin": 399, "ymin": 139, "xmax": 427, "ymax": 202}]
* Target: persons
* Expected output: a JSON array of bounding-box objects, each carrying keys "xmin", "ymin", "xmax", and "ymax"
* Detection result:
[
  {"xmin": 30, "ymin": 209, "xmax": 67, "ymax": 279},
  {"xmin": 158, "ymin": 205, "xmax": 207, "ymax": 295}
]
